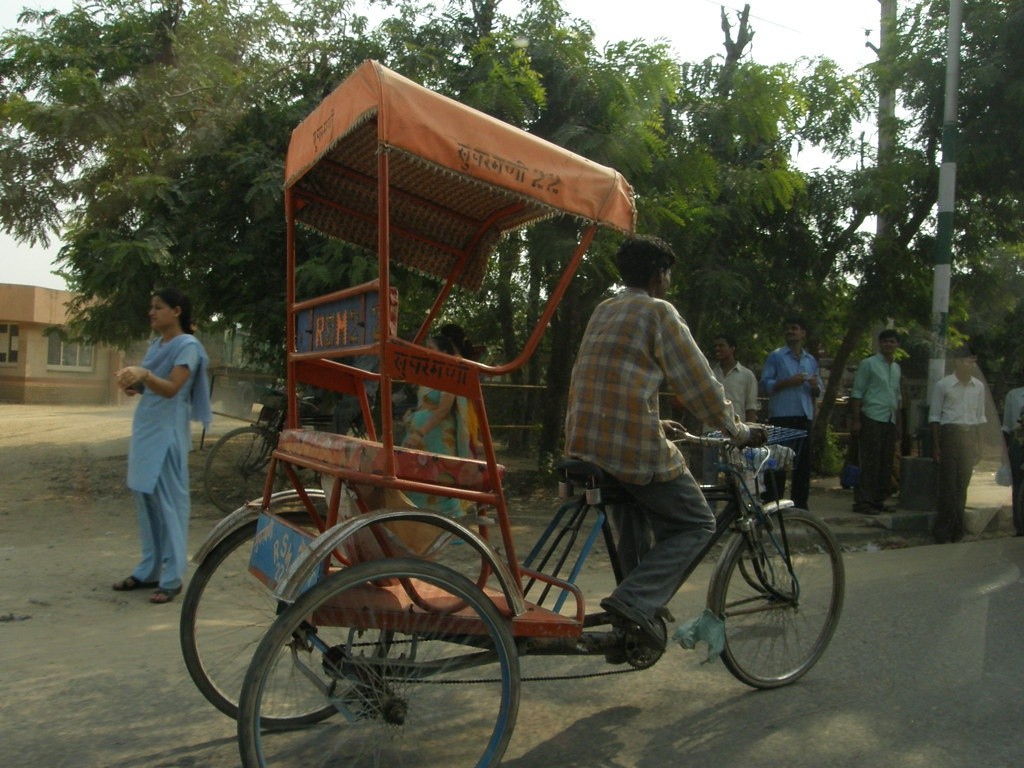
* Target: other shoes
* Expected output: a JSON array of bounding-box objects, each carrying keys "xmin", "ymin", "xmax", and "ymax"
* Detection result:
[
  {"xmin": 879, "ymin": 504, "xmax": 896, "ymax": 512},
  {"xmin": 861, "ymin": 508, "xmax": 881, "ymax": 514},
  {"xmin": 599, "ymin": 594, "xmax": 666, "ymax": 653}
]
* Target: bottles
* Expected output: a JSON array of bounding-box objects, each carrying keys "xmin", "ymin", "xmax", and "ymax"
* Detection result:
[{"xmin": 725, "ymin": 444, "xmax": 777, "ymax": 471}]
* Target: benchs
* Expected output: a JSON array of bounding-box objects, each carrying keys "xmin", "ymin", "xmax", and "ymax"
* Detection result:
[{"xmin": 271, "ymin": 281, "xmax": 523, "ymax": 611}]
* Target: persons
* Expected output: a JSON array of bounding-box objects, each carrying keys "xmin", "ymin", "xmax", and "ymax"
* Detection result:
[
  {"xmin": 703, "ymin": 334, "xmax": 759, "ymax": 512},
  {"xmin": 333, "ymin": 353, "xmax": 380, "ymax": 435},
  {"xmin": 114, "ymin": 289, "xmax": 213, "ymax": 603},
  {"xmin": 840, "ymin": 354, "xmax": 876, "ymax": 489},
  {"xmin": 930, "ymin": 347, "xmax": 986, "ymax": 544},
  {"xmin": 1001, "ymin": 386, "xmax": 1024, "ymax": 537},
  {"xmin": 399, "ymin": 326, "xmax": 474, "ymax": 543},
  {"xmin": 760, "ymin": 319, "xmax": 822, "ymax": 510},
  {"xmin": 852, "ymin": 329, "xmax": 903, "ymax": 515},
  {"xmin": 565, "ymin": 236, "xmax": 767, "ymax": 664}
]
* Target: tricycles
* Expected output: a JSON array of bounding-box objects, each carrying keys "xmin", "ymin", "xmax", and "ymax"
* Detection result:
[{"xmin": 178, "ymin": 56, "xmax": 849, "ymax": 768}]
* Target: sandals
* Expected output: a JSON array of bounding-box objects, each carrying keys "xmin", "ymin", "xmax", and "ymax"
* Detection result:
[
  {"xmin": 113, "ymin": 576, "xmax": 160, "ymax": 591},
  {"xmin": 149, "ymin": 584, "xmax": 182, "ymax": 603}
]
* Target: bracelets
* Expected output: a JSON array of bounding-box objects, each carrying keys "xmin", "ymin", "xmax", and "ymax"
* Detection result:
[
  {"xmin": 416, "ymin": 430, "xmax": 423, "ymax": 437},
  {"xmin": 143, "ymin": 370, "xmax": 150, "ymax": 381}
]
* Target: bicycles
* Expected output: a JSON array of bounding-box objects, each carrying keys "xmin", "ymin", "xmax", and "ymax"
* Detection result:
[{"xmin": 203, "ymin": 373, "xmax": 420, "ymax": 513}]
